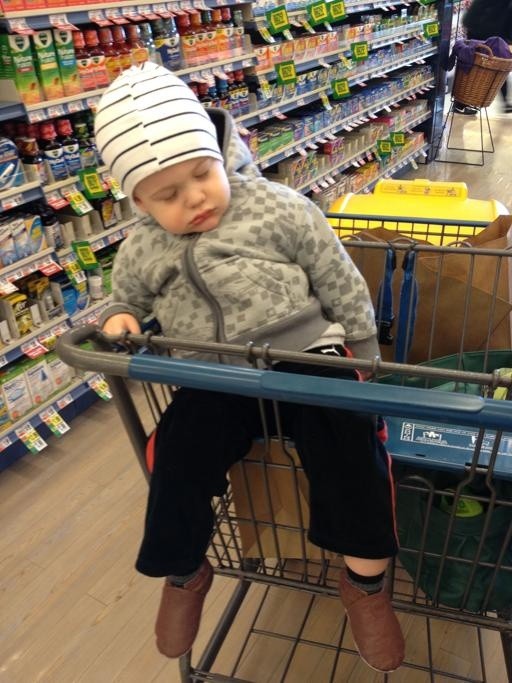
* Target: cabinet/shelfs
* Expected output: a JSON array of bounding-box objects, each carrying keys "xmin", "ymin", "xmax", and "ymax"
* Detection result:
[{"xmin": 1, "ymin": 0, "xmax": 454, "ymax": 476}]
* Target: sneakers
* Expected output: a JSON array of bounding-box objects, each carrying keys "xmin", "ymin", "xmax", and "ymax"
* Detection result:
[
  {"xmin": 451, "ymin": 105, "xmax": 478, "ymax": 117},
  {"xmin": 338, "ymin": 566, "xmax": 406, "ymax": 674},
  {"xmin": 154, "ymin": 555, "xmax": 213, "ymax": 659}
]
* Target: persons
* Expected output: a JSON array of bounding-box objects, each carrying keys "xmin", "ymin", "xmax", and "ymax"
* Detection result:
[
  {"xmin": 92, "ymin": 60, "xmax": 408, "ymax": 673},
  {"xmin": 453, "ymin": 1, "xmax": 512, "ymax": 117}
]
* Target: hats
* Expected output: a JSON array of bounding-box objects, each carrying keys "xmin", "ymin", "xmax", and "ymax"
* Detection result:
[{"xmin": 94, "ymin": 60, "xmax": 225, "ymax": 212}]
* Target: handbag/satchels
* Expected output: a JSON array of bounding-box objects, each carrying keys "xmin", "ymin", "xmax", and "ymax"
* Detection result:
[
  {"xmin": 366, "ymin": 349, "xmax": 512, "ymax": 613},
  {"xmin": 340, "ymin": 213, "xmax": 512, "ymax": 375}
]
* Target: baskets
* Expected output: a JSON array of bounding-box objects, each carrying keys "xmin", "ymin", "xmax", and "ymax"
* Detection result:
[{"xmin": 453, "ymin": 40, "xmax": 512, "ymax": 107}]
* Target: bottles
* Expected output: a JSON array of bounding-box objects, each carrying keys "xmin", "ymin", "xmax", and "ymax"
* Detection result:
[{"xmin": 19, "ymin": 6, "xmax": 246, "ymax": 253}]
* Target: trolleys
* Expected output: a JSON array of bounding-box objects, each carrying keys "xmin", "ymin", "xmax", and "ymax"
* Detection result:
[{"xmin": 56, "ymin": 212, "xmax": 512, "ymax": 683}]
